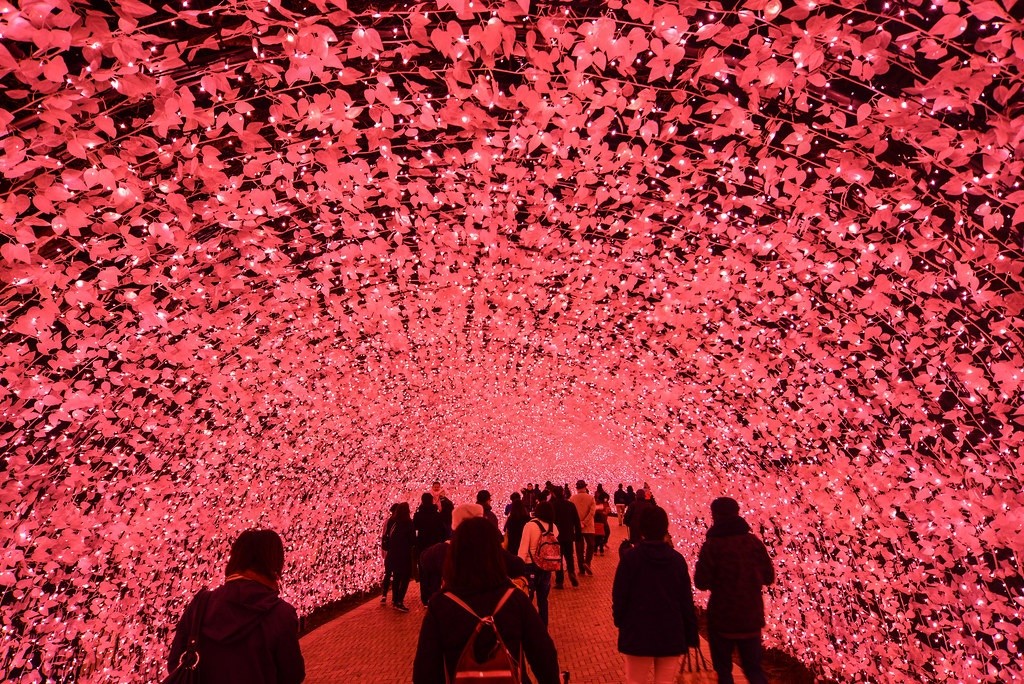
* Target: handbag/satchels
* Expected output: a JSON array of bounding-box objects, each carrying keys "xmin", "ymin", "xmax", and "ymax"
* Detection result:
[
  {"xmin": 593, "ymin": 523, "xmax": 604, "ymax": 535},
  {"xmin": 382, "ymin": 529, "xmax": 389, "ymax": 551},
  {"xmin": 161, "ymin": 593, "xmax": 210, "ymax": 684},
  {"xmin": 677, "ymin": 646, "xmax": 718, "ymax": 684}
]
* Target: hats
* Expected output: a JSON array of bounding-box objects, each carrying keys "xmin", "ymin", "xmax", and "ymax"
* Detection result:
[
  {"xmin": 451, "ymin": 504, "xmax": 484, "ymax": 530},
  {"xmin": 575, "ymin": 480, "xmax": 588, "ymax": 489}
]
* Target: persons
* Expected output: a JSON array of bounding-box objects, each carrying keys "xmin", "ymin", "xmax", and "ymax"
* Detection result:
[
  {"xmin": 696, "ymin": 497, "xmax": 775, "ymax": 683},
  {"xmin": 517, "ymin": 501, "xmax": 563, "ymax": 629},
  {"xmin": 502, "ymin": 491, "xmax": 529, "ymax": 554},
  {"xmin": 419, "ymin": 502, "xmax": 527, "ymax": 610},
  {"xmin": 520, "ymin": 480, "xmax": 657, "ymax": 589},
  {"xmin": 411, "ymin": 492, "xmax": 454, "ymax": 577},
  {"xmin": 161, "ymin": 528, "xmax": 306, "ymax": 684},
  {"xmin": 611, "ymin": 507, "xmax": 699, "ymax": 684},
  {"xmin": 425, "ymin": 480, "xmax": 446, "ymax": 514},
  {"xmin": 380, "ymin": 502, "xmax": 414, "ymax": 613},
  {"xmin": 470, "ymin": 489, "xmax": 504, "ymax": 553},
  {"xmin": 412, "ymin": 516, "xmax": 561, "ymax": 684}
]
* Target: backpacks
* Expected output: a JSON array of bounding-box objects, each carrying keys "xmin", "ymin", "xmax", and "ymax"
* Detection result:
[
  {"xmin": 441, "ymin": 587, "xmax": 539, "ymax": 684},
  {"xmin": 528, "ymin": 520, "xmax": 561, "ymax": 571}
]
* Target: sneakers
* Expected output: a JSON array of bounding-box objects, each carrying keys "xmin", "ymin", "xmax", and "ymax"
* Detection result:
[
  {"xmin": 583, "ymin": 563, "xmax": 593, "ymax": 575},
  {"xmin": 394, "ymin": 604, "xmax": 409, "ymax": 612},
  {"xmin": 569, "ymin": 574, "xmax": 578, "ymax": 586},
  {"xmin": 552, "ymin": 584, "xmax": 563, "ymax": 590},
  {"xmin": 379, "ymin": 598, "xmax": 386, "ymax": 604}
]
